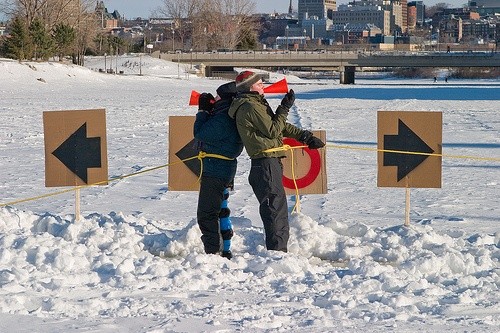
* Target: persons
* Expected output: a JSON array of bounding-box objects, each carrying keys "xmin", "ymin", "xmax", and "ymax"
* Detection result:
[
  {"xmin": 229, "ymin": 70, "xmax": 325, "ymax": 253},
  {"xmin": 434, "ymin": 74, "xmax": 437, "ymax": 82},
  {"xmin": 446, "ymin": 43, "xmax": 450, "ymax": 53},
  {"xmin": 445, "ymin": 73, "xmax": 448, "ymax": 83},
  {"xmin": 193, "ymin": 81, "xmax": 244, "ymax": 260}
]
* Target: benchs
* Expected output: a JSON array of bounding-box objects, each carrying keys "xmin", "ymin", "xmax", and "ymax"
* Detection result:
[
  {"xmin": 99, "ymin": 69, "xmax": 105, "ymax": 73},
  {"xmin": 107, "ymin": 69, "xmax": 115, "ymax": 74}
]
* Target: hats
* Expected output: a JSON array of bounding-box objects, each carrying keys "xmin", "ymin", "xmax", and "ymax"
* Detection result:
[
  {"xmin": 216, "ymin": 81, "xmax": 236, "ymax": 100},
  {"xmin": 235, "ymin": 70, "xmax": 263, "ymax": 90}
]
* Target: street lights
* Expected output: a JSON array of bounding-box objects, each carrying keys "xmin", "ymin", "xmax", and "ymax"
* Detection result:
[
  {"xmin": 286, "ymin": 25, "xmax": 290, "ymax": 50},
  {"xmin": 172, "ymin": 31, "xmax": 175, "ymax": 51},
  {"xmin": 437, "ymin": 29, "xmax": 440, "ymax": 51},
  {"xmin": 189, "ymin": 48, "xmax": 193, "ymax": 68},
  {"xmin": 429, "ymin": 25, "xmax": 433, "ymax": 50}
]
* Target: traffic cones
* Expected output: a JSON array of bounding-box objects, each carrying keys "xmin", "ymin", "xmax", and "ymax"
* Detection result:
[
  {"xmin": 189, "ymin": 91, "xmax": 215, "ymax": 106},
  {"xmin": 262, "ymin": 76, "xmax": 289, "ymax": 94}
]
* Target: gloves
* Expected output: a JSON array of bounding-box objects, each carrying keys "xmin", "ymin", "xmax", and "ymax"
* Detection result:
[
  {"xmin": 301, "ymin": 130, "xmax": 326, "ymax": 150},
  {"xmin": 198, "ymin": 93, "xmax": 210, "ymax": 112},
  {"xmin": 207, "ymin": 92, "xmax": 215, "ymax": 105},
  {"xmin": 280, "ymin": 88, "xmax": 296, "ymax": 109}
]
